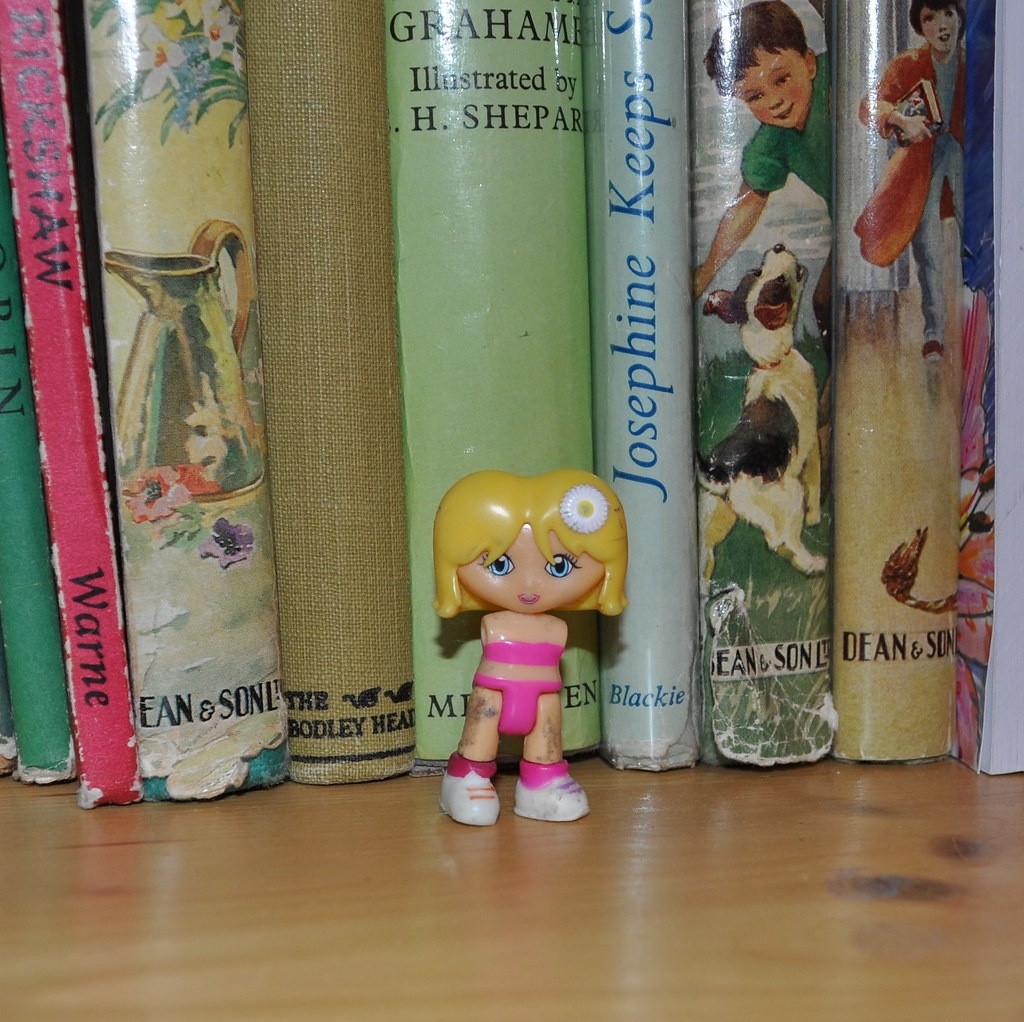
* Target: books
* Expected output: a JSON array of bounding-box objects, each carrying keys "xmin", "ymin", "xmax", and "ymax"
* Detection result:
[{"xmin": 1, "ymin": 0, "xmax": 1024, "ymax": 810}]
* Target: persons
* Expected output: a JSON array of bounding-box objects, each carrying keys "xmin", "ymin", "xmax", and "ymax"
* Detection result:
[{"xmin": 432, "ymin": 469, "xmax": 629, "ymax": 826}]
